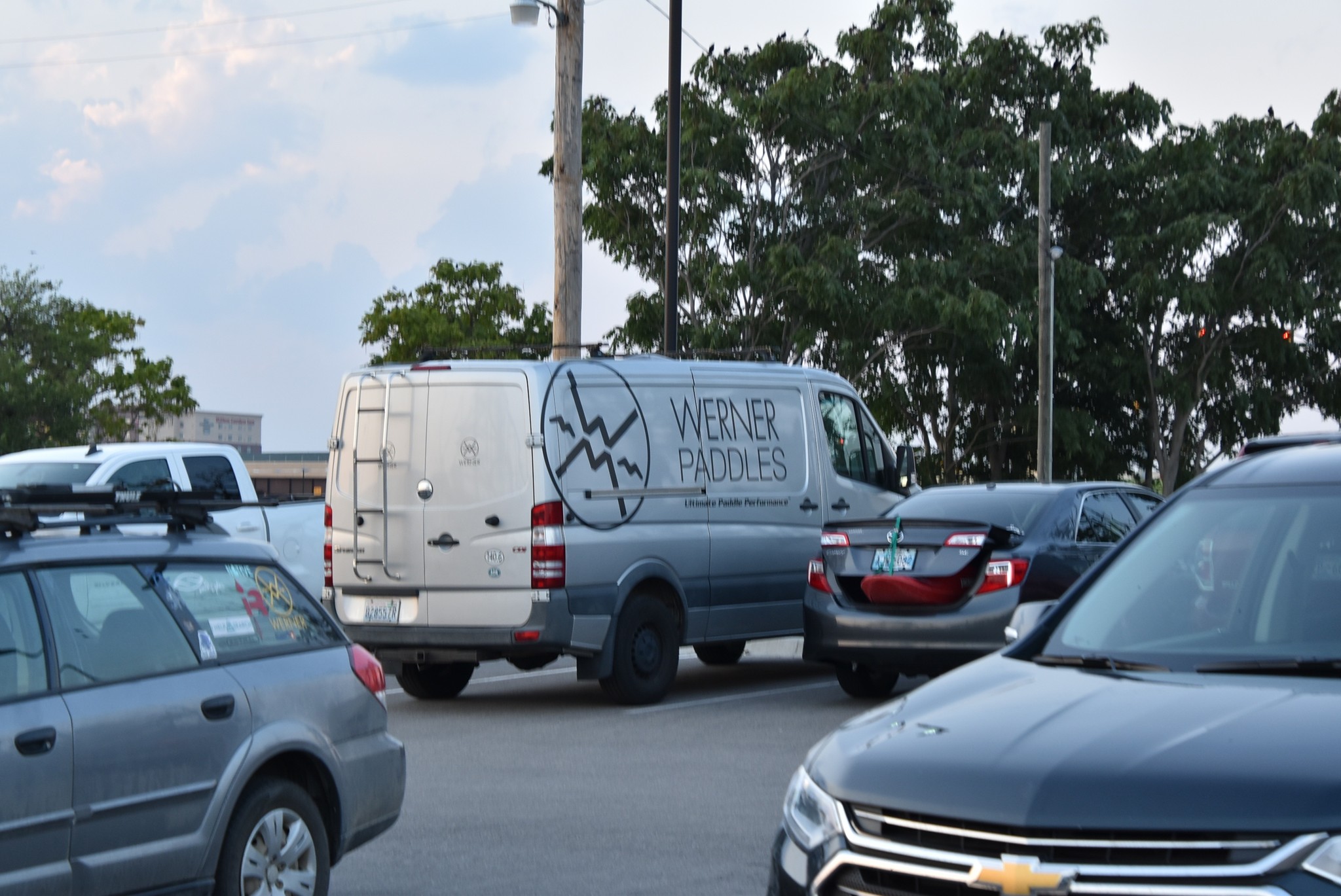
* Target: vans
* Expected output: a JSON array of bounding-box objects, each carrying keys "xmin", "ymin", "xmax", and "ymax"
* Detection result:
[
  {"xmin": 0, "ymin": 428, "xmax": 271, "ymax": 617},
  {"xmin": 320, "ymin": 344, "xmax": 930, "ymax": 706}
]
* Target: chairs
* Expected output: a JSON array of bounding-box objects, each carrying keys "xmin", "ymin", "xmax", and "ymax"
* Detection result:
[{"xmin": 92, "ymin": 607, "xmax": 187, "ymax": 681}]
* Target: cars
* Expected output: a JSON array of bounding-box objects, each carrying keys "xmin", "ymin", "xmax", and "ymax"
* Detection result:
[
  {"xmin": 804, "ymin": 481, "xmax": 1170, "ymax": 703},
  {"xmin": 768, "ymin": 436, "xmax": 1341, "ymax": 896}
]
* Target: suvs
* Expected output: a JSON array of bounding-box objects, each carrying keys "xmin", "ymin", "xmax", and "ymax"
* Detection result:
[{"xmin": 0, "ymin": 478, "xmax": 405, "ymax": 896}]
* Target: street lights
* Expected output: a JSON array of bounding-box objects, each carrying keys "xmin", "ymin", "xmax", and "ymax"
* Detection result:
[{"xmin": 511, "ymin": 1, "xmax": 583, "ymax": 368}]
